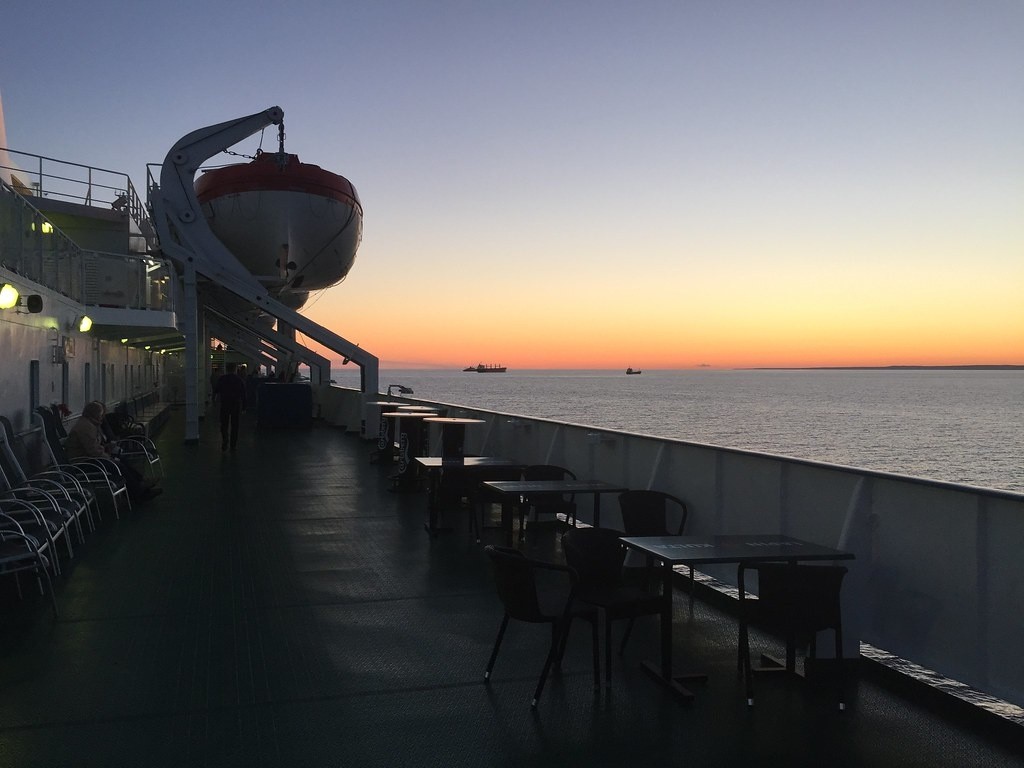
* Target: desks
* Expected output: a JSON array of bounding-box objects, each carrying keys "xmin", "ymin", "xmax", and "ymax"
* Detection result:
[
  {"xmin": 367, "ymin": 402, "xmax": 486, "ymax": 502},
  {"xmin": 415, "ymin": 457, "xmax": 528, "ymax": 537},
  {"xmin": 619, "ymin": 534, "xmax": 857, "ymax": 702},
  {"xmin": 480, "ymin": 480, "xmax": 630, "ymax": 547}
]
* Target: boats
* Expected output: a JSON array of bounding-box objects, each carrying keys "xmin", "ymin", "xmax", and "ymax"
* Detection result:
[
  {"xmin": 463, "ymin": 366, "xmax": 477, "ymax": 372},
  {"xmin": 194, "ymin": 150, "xmax": 363, "ymax": 327},
  {"xmin": 477, "ymin": 362, "xmax": 507, "ymax": 373},
  {"xmin": 626, "ymin": 366, "xmax": 642, "ymax": 374}
]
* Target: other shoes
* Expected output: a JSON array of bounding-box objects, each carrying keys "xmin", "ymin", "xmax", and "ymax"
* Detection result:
[
  {"xmin": 148, "ymin": 484, "xmax": 162, "ymax": 496},
  {"xmin": 230, "ymin": 446, "xmax": 239, "ymax": 451},
  {"xmin": 222, "ymin": 439, "xmax": 229, "ymax": 451}
]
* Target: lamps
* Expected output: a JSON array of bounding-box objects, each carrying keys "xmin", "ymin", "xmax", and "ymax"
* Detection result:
[
  {"xmin": 67, "ymin": 315, "xmax": 92, "ymax": 332},
  {"xmin": 0, "ymin": 283, "xmax": 43, "ymax": 314}
]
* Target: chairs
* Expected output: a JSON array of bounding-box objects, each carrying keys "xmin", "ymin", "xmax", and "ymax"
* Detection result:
[
  {"xmin": 440, "ymin": 463, "xmax": 688, "ymax": 708},
  {"xmin": 738, "ymin": 560, "xmax": 847, "ymax": 713},
  {"xmin": 0, "ymin": 391, "xmax": 171, "ymax": 616}
]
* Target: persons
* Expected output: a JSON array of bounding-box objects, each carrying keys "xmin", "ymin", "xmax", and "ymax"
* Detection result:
[
  {"xmin": 64, "ymin": 401, "xmax": 163, "ymax": 492},
  {"xmin": 211, "ymin": 368, "xmax": 220, "ymax": 401},
  {"xmin": 217, "ymin": 343, "xmax": 222, "ymax": 351},
  {"xmin": 214, "ymin": 363, "xmax": 247, "ymax": 451}
]
